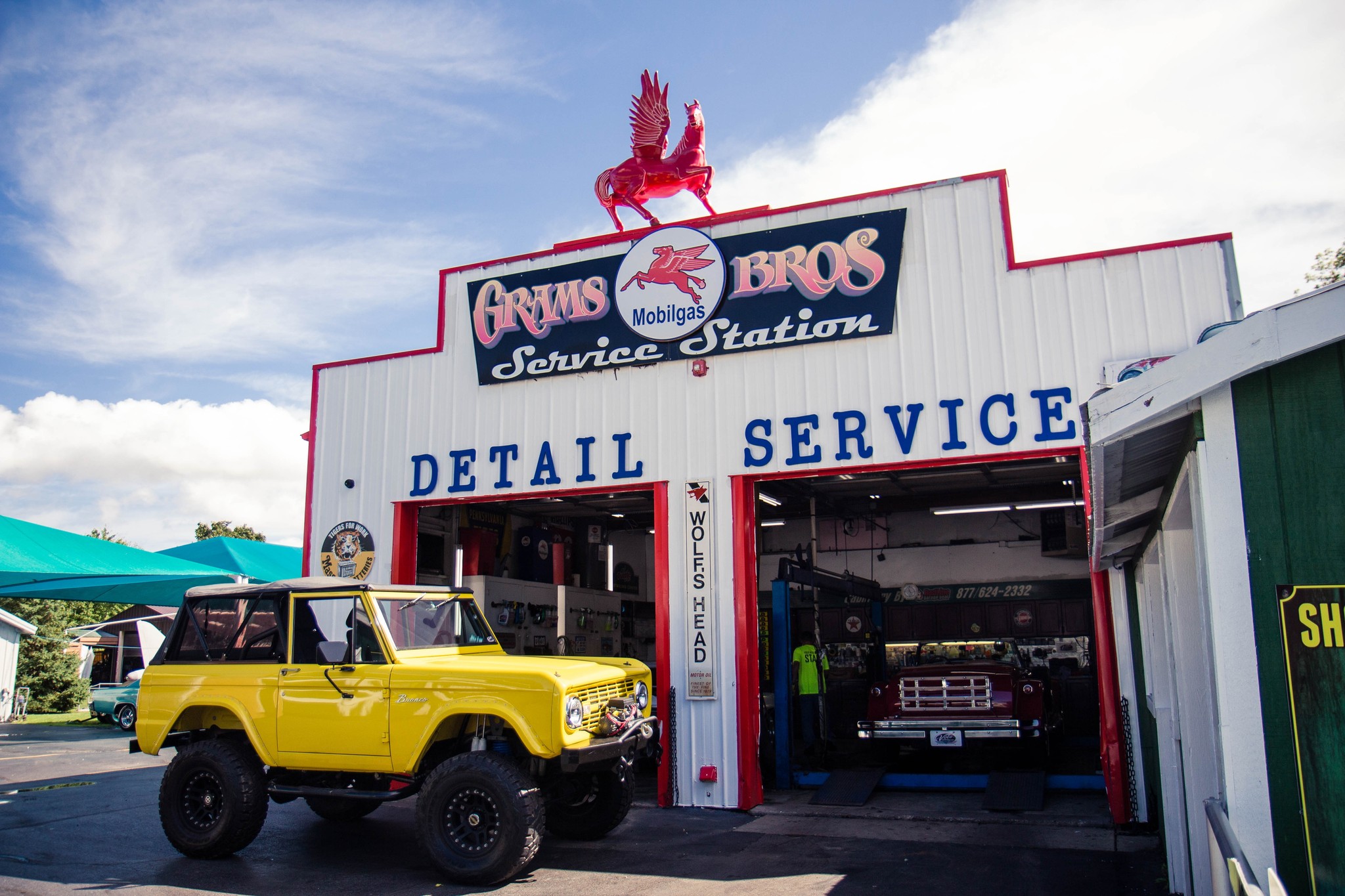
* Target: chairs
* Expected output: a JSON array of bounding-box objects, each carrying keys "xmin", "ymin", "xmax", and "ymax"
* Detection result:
[{"xmin": 280, "ymin": 601, "xmax": 330, "ymax": 663}]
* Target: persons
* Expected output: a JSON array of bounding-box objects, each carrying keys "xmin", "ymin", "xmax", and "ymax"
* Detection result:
[{"xmin": 793, "ymin": 632, "xmax": 829, "ymax": 752}]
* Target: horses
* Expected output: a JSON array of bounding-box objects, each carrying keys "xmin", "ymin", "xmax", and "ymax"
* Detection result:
[{"xmin": 593, "ymin": 70, "xmax": 715, "ymax": 233}]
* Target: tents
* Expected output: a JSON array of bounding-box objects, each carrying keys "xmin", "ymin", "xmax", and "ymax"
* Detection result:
[
  {"xmin": 155, "ymin": 538, "xmax": 302, "ymax": 583},
  {"xmin": 0, "ymin": 513, "xmax": 251, "ymax": 607}
]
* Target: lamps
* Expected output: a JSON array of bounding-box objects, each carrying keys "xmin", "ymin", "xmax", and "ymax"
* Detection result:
[
  {"xmin": 605, "ymin": 542, "xmax": 614, "ymax": 592},
  {"xmin": 452, "ymin": 543, "xmax": 462, "ymax": 637},
  {"xmin": 930, "ymin": 497, "xmax": 1084, "ymax": 515},
  {"xmin": 761, "ymin": 518, "xmax": 787, "ymax": 526},
  {"xmin": 759, "ymin": 491, "xmax": 782, "ymax": 507}
]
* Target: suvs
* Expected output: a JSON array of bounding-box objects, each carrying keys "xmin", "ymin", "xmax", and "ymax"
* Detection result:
[
  {"xmin": 130, "ymin": 577, "xmax": 663, "ymax": 885},
  {"xmin": 858, "ymin": 639, "xmax": 1045, "ymax": 770}
]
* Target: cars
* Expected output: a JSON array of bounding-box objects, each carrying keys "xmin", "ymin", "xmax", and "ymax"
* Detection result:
[{"xmin": 89, "ymin": 678, "xmax": 140, "ymax": 731}]
